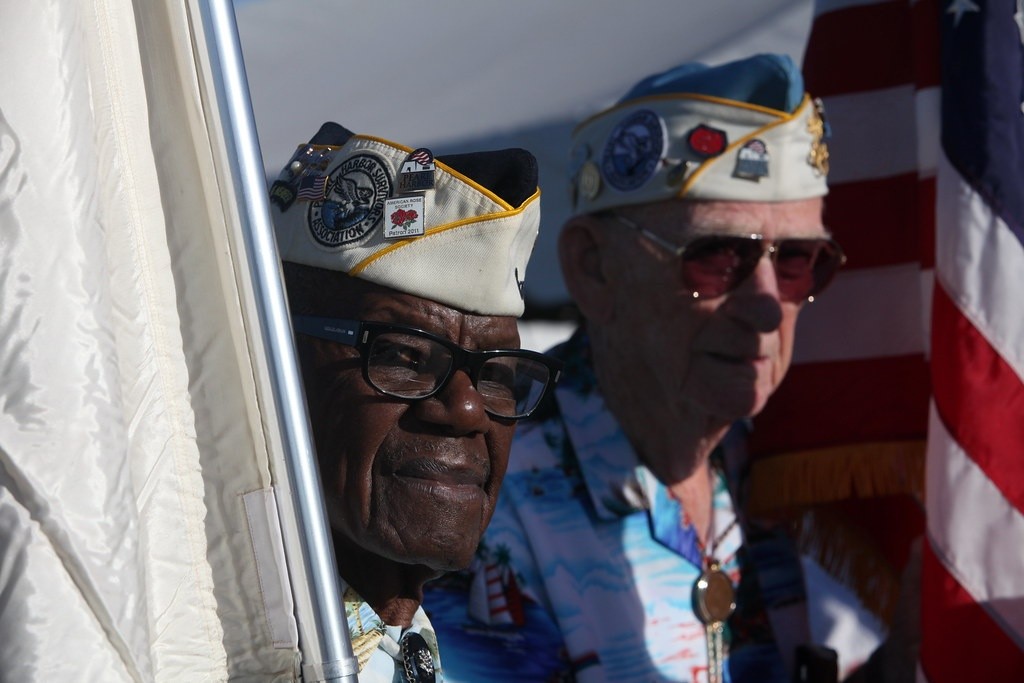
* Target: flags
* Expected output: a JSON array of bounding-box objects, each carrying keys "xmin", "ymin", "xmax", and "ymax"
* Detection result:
[
  {"xmin": 0, "ymin": 0, "xmax": 303, "ymax": 683},
  {"xmin": 740, "ymin": 0, "xmax": 1024, "ymax": 683}
]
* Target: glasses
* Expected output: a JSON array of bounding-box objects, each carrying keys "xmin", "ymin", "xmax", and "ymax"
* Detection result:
[
  {"xmin": 601, "ymin": 209, "xmax": 849, "ymax": 304},
  {"xmin": 292, "ymin": 315, "xmax": 560, "ymax": 419}
]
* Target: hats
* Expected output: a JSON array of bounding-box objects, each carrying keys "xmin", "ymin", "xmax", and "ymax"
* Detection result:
[
  {"xmin": 269, "ymin": 120, "xmax": 540, "ymax": 317},
  {"xmin": 569, "ymin": 52, "xmax": 831, "ymax": 216}
]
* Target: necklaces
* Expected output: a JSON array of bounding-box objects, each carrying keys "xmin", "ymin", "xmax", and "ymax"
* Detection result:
[
  {"xmin": 401, "ymin": 634, "xmax": 436, "ymax": 683},
  {"xmin": 695, "ymin": 514, "xmax": 740, "ymax": 683}
]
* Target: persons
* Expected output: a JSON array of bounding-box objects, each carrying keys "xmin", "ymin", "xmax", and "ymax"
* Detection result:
[
  {"xmin": 420, "ymin": 55, "xmax": 844, "ymax": 683},
  {"xmin": 269, "ymin": 121, "xmax": 561, "ymax": 683}
]
